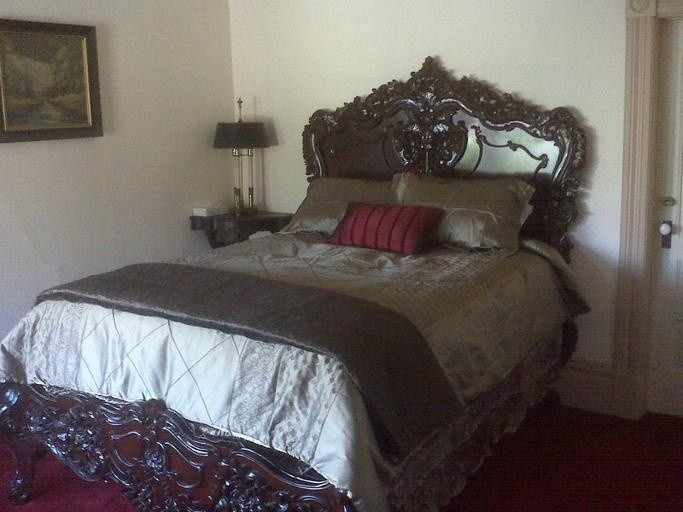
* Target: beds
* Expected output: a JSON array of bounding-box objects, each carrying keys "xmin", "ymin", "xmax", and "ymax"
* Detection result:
[{"xmin": 0, "ymin": 56, "xmax": 589, "ymax": 512}]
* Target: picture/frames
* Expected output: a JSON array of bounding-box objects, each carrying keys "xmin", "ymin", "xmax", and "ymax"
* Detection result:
[{"xmin": 0, "ymin": 18, "xmax": 103, "ymax": 143}]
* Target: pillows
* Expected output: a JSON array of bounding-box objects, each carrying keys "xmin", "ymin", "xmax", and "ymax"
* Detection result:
[
  {"xmin": 391, "ymin": 173, "xmax": 536, "ymax": 257},
  {"xmin": 327, "ymin": 202, "xmax": 442, "ymax": 254},
  {"xmin": 280, "ymin": 176, "xmax": 391, "ymax": 235}
]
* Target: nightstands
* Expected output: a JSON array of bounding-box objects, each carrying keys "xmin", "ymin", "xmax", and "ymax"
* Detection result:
[{"xmin": 190, "ymin": 212, "xmax": 293, "ymax": 250}]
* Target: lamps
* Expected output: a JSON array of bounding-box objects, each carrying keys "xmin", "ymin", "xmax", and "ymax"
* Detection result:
[{"xmin": 213, "ymin": 97, "xmax": 269, "ymax": 216}]
